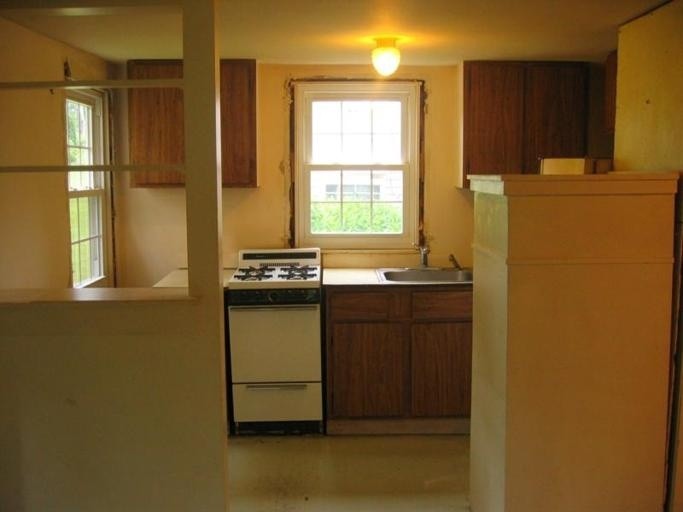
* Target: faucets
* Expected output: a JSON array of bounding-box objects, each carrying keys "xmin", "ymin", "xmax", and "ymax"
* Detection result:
[
  {"xmin": 448, "ymin": 253, "xmax": 463, "ymax": 270},
  {"xmin": 421, "ymin": 246, "xmax": 431, "ymax": 266}
]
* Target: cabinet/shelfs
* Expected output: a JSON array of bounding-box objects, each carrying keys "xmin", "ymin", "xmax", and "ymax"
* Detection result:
[
  {"xmin": 463, "ymin": 59, "xmax": 588, "ymax": 183},
  {"xmin": 126, "ymin": 56, "xmax": 257, "ymax": 187},
  {"xmin": 460, "ymin": 169, "xmax": 677, "ymax": 511},
  {"xmin": 604, "ymin": 51, "xmax": 619, "ymax": 138},
  {"xmin": 328, "ymin": 291, "xmax": 472, "ymax": 419}
]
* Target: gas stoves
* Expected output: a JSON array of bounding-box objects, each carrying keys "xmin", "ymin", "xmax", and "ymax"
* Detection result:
[{"xmin": 225, "ymin": 261, "xmax": 322, "ymax": 305}]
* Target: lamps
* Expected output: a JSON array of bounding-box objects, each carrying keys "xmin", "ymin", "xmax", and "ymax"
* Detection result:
[{"xmin": 369, "ymin": 36, "xmax": 401, "ymax": 76}]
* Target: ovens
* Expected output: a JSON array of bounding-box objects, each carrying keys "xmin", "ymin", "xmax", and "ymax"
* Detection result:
[{"xmin": 223, "ymin": 304, "xmax": 323, "ymax": 438}]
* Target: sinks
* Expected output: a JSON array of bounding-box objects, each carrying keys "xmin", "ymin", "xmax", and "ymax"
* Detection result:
[{"xmin": 380, "ymin": 267, "xmax": 474, "ymax": 284}]
